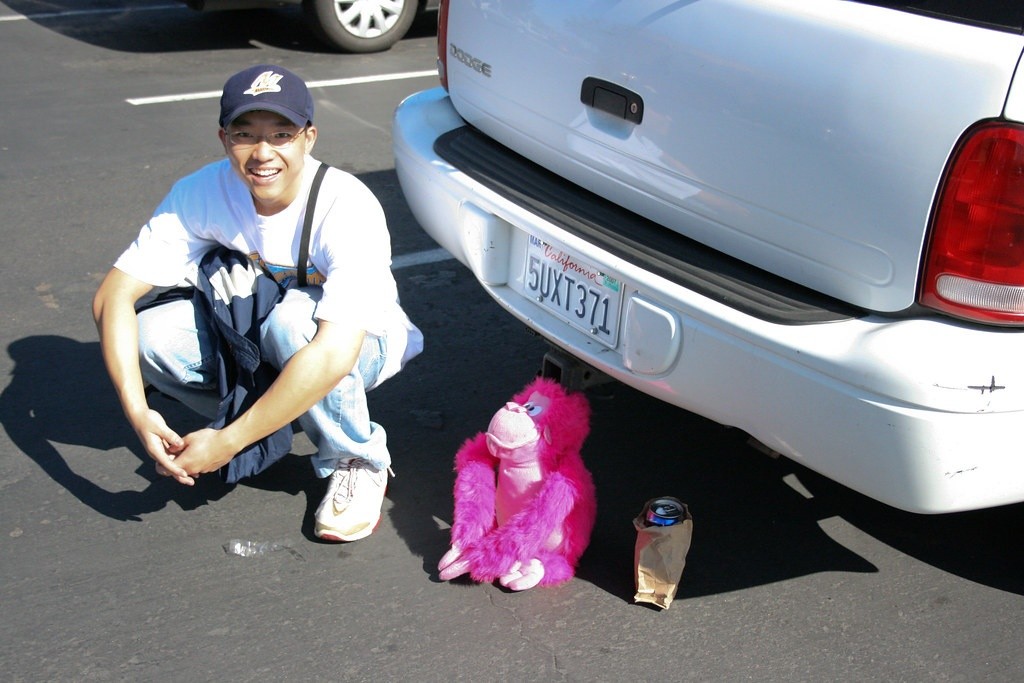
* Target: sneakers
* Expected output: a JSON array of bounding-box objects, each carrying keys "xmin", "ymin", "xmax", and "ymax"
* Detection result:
[{"xmin": 314, "ymin": 458, "xmax": 397, "ymax": 543}]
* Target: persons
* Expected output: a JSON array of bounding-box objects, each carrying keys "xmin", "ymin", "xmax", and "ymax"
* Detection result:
[{"xmin": 92, "ymin": 64, "xmax": 424, "ymax": 542}]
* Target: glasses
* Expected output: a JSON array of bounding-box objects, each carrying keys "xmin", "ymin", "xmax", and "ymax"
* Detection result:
[{"xmin": 224, "ymin": 126, "xmax": 309, "ymax": 144}]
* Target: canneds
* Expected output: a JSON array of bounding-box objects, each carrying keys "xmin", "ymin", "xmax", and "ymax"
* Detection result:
[{"xmin": 644, "ymin": 499, "xmax": 684, "ymax": 526}]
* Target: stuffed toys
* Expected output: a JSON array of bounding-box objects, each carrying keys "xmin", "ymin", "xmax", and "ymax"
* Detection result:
[{"xmin": 437, "ymin": 377, "xmax": 595, "ymax": 590}]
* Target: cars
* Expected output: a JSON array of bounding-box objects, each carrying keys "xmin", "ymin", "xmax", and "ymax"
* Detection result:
[{"xmin": 388, "ymin": 0, "xmax": 1024, "ymax": 516}]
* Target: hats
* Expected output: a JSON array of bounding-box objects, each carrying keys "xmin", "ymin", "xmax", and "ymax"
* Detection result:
[{"xmin": 218, "ymin": 65, "xmax": 314, "ymax": 130}]
